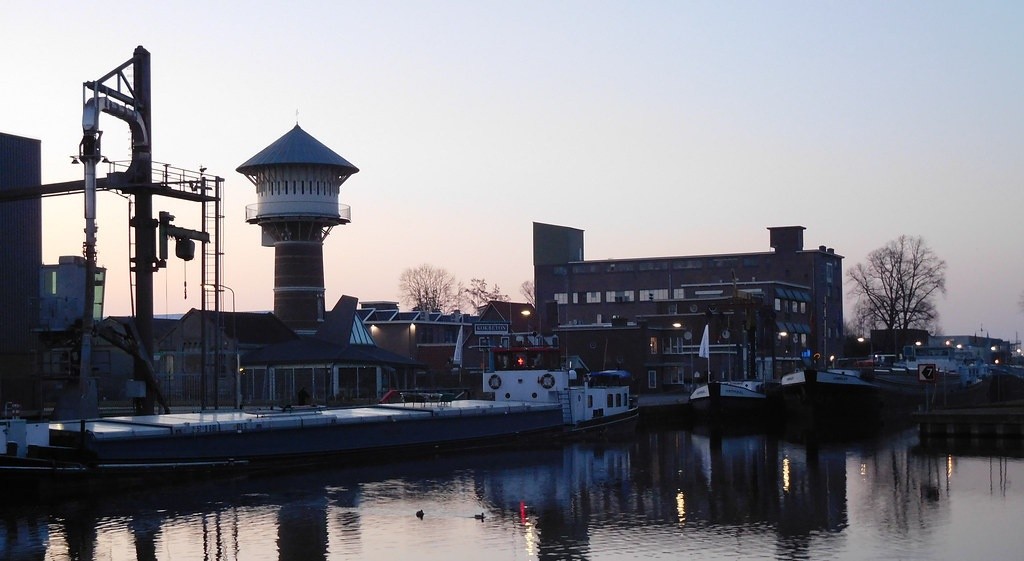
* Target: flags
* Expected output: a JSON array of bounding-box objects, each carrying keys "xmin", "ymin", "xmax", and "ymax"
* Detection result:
[
  {"xmin": 453, "ymin": 326, "xmax": 462, "ymax": 365},
  {"xmin": 698, "ymin": 324, "xmax": 709, "ymax": 359}
]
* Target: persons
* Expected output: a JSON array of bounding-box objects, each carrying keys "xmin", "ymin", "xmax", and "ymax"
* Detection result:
[{"xmin": 297, "ymin": 384, "xmax": 309, "ymax": 406}]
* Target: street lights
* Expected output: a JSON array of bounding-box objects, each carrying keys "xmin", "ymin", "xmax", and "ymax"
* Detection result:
[
  {"xmin": 672, "ymin": 323, "xmax": 694, "ymax": 386},
  {"xmin": 912, "ymin": 342, "xmax": 921, "ymax": 361},
  {"xmin": 521, "ymin": 310, "xmax": 541, "ymax": 346},
  {"xmin": 945, "ymin": 341, "xmax": 963, "ymax": 359},
  {"xmin": 772, "ymin": 330, "xmax": 788, "ymax": 378},
  {"xmin": 857, "ymin": 338, "xmax": 875, "ymax": 369}
]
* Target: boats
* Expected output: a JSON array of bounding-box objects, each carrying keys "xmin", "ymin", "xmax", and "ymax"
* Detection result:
[
  {"xmin": 0, "ymin": 333, "xmax": 642, "ymax": 478},
  {"xmin": 688, "ymin": 341, "xmax": 1024, "ymax": 418},
  {"xmin": 244, "ymin": 405, "xmax": 328, "ymax": 413}
]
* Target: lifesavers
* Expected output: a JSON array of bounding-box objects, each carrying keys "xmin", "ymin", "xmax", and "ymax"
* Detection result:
[
  {"xmin": 541, "ymin": 374, "xmax": 555, "ymax": 388},
  {"xmin": 489, "ymin": 374, "xmax": 501, "ymax": 389}
]
